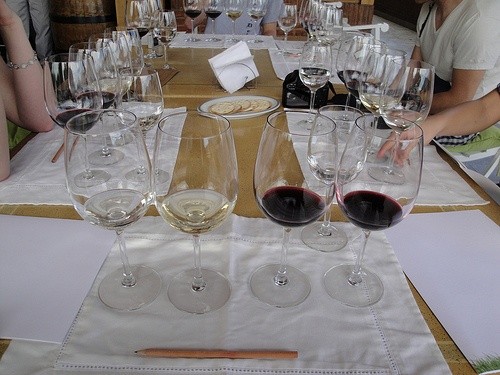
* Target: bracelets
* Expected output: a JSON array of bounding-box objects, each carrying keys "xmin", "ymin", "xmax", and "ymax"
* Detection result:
[{"xmin": 9, "ymin": 50, "xmax": 37, "ymax": 69}]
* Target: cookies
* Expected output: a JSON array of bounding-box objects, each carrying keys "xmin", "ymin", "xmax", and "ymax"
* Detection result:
[{"xmin": 208, "ymin": 99, "xmax": 272, "ymax": 115}]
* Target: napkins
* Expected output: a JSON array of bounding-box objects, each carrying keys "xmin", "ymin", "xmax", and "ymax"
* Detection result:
[{"xmin": 206, "ymin": 40, "xmax": 259, "ymax": 93}]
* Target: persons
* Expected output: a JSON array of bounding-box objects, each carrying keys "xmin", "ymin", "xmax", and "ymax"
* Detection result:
[
  {"xmin": 377, "ymin": 81, "xmax": 500, "ymax": 164},
  {"xmin": 0, "ymin": 0, "xmax": 57, "ymax": 181},
  {"xmin": 384, "ymin": 0, "xmax": 500, "ymax": 147},
  {"xmin": 6, "ymin": 0, "xmax": 51, "ymax": 67},
  {"xmin": 185, "ymin": 0, "xmax": 284, "ymax": 37}
]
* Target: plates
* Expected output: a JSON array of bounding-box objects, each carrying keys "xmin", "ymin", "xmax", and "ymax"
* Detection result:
[{"xmin": 196, "ymin": 94, "xmax": 280, "ymax": 120}]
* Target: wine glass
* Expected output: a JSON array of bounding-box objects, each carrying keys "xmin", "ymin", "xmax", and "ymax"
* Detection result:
[
  {"xmin": 42, "ymin": 0, "xmax": 177, "ymax": 188},
  {"xmin": 299, "ymin": 0, "xmax": 436, "ymax": 185},
  {"xmin": 248, "ymin": 109, "xmax": 338, "ymax": 309},
  {"xmin": 63, "ymin": 107, "xmax": 163, "ymax": 313},
  {"xmin": 299, "ymin": 40, "xmax": 333, "ymax": 133},
  {"xmin": 223, "ymin": 0, "xmax": 245, "ymax": 42},
  {"xmin": 204, "ymin": 0, "xmax": 223, "ymax": 43},
  {"xmin": 245, "ymin": 0, "xmax": 269, "ymax": 44},
  {"xmin": 300, "ymin": 104, "xmax": 368, "ymax": 254},
  {"xmin": 318, "ymin": 114, "xmax": 424, "ymax": 309},
  {"xmin": 182, "ymin": 0, "xmax": 204, "ymax": 42},
  {"xmin": 277, "ymin": 2, "xmax": 298, "ymax": 57},
  {"xmin": 151, "ymin": 110, "xmax": 240, "ymax": 314}
]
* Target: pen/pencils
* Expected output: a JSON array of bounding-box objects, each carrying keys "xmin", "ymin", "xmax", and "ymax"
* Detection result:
[
  {"xmin": 135, "ymin": 348, "xmax": 300, "ymax": 360},
  {"xmin": 52, "ymin": 144, "xmax": 64, "ymax": 163}
]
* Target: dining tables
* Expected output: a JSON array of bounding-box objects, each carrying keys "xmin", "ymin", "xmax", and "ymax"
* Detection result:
[{"xmin": 0, "ymin": 29, "xmax": 500, "ymax": 375}]
